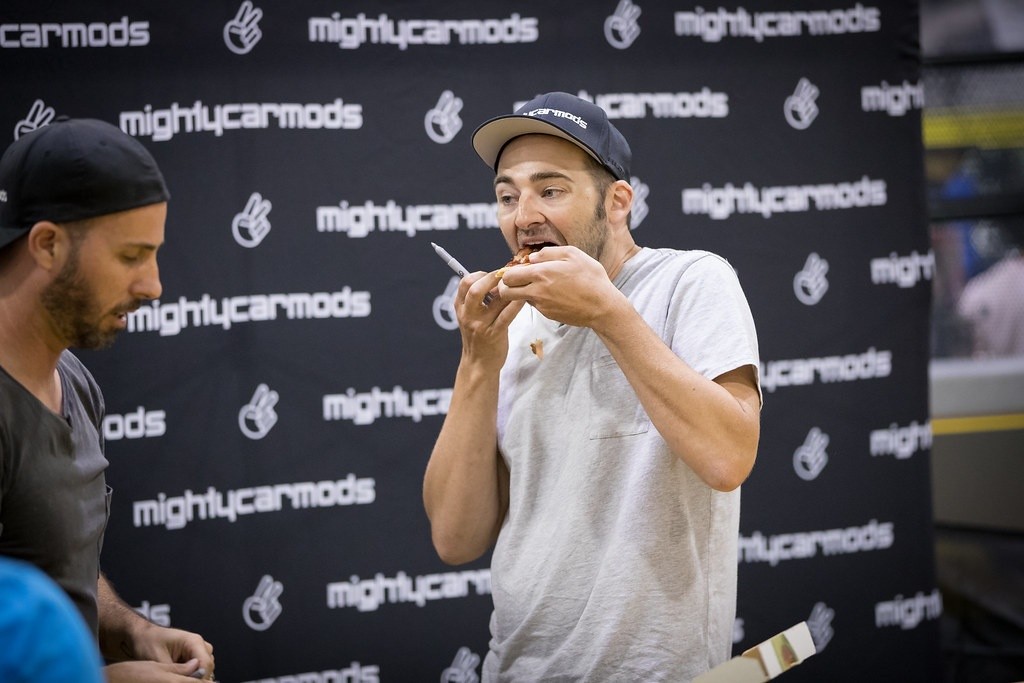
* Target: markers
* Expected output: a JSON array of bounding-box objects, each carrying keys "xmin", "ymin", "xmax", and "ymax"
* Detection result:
[{"xmin": 430, "ymin": 241, "xmax": 495, "ymax": 304}]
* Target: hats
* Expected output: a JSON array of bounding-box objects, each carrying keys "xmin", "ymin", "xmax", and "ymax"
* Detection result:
[
  {"xmin": 0, "ymin": 117, "xmax": 170, "ymax": 246},
  {"xmin": 474, "ymin": 92, "xmax": 631, "ymax": 185}
]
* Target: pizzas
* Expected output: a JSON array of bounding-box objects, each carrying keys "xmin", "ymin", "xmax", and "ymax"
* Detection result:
[{"xmin": 495, "ymin": 247, "xmax": 538, "ymax": 278}]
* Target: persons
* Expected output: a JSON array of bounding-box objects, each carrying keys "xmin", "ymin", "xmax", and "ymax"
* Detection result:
[
  {"xmin": 0, "ymin": 117, "xmax": 214, "ymax": 683},
  {"xmin": 423, "ymin": 93, "xmax": 763, "ymax": 683}
]
